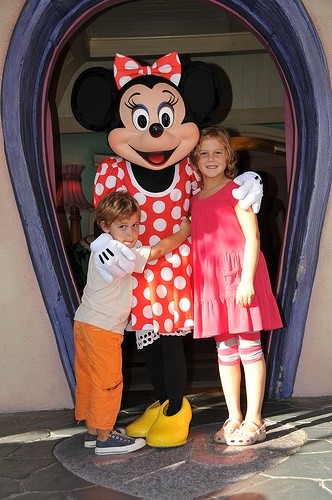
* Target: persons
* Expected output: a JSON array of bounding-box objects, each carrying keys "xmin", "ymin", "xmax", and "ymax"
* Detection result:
[
  {"xmin": 74, "ymin": 190, "xmax": 192, "ymax": 455},
  {"xmin": 188, "ymin": 124, "xmax": 284, "ymax": 446}
]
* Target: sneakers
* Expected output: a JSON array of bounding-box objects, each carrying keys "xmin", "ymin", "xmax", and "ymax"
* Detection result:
[{"xmin": 83, "ymin": 427, "xmax": 148, "ymax": 457}]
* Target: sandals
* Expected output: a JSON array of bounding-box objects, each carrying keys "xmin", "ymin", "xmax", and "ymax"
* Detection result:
[{"xmin": 213, "ymin": 417, "xmax": 268, "ymax": 447}]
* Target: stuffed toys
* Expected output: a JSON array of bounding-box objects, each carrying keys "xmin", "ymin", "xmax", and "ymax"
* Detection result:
[{"xmin": 70, "ymin": 51, "xmax": 263, "ymax": 448}]
